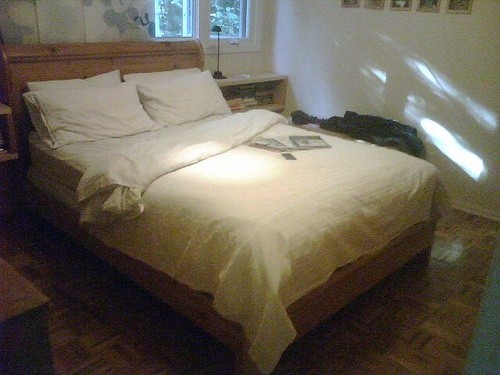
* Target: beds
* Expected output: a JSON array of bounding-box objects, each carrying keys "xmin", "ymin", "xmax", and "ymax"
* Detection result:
[{"xmin": 0, "ymin": 36, "xmax": 437, "ymax": 375}]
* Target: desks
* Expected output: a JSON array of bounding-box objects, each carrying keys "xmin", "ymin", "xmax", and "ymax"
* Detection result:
[{"xmin": 0, "ymin": 256, "xmax": 53, "ymax": 375}]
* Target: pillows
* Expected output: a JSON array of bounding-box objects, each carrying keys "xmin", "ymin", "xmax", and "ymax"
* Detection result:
[
  {"xmin": 27, "ymin": 69, "xmax": 122, "ymax": 89},
  {"xmin": 21, "ymin": 79, "xmax": 162, "ymax": 148},
  {"xmin": 135, "ymin": 70, "xmax": 231, "ymax": 128},
  {"xmin": 124, "ymin": 67, "xmax": 202, "ymax": 83}
]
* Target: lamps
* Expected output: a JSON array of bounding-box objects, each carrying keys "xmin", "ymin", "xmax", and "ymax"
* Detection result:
[{"xmin": 211, "ymin": 25, "xmax": 226, "ymax": 79}]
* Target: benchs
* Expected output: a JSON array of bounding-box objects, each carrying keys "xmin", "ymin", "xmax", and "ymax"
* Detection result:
[{"xmin": 288, "ymin": 115, "xmax": 400, "ymax": 150}]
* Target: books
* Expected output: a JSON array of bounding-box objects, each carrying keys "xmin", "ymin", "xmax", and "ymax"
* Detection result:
[
  {"xmin": 247, "ymin": 134, "xmax": 333, "ymax": 153},
  {"xmin": 226, "ymin": 83, "xmax": 282, "ymax": 114}
]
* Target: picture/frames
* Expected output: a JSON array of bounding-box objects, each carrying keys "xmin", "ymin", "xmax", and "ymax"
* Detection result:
[
  {"xmin": 364, "ymin": 0, "xmax": 385, "ymax": 10},
  {"xmin": 389, "ymin": 0, "xmax": 412, "ymax": 11},
  {"xmin": 445, "ymin": 0, "xmax": 473, "ymax": 14},
  {"xmin": 415, "ymin": 0, "xmax": 441, "ymax": 13},
  {"xmin": 341, "ymin": 0, "xmax": 361, "ymax": 8}
]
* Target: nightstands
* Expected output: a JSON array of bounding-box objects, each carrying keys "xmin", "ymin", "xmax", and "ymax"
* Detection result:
[
  {"xmin": 215, "ymin": 70, "xmax": 288, "ymax": 113},
  {"xmin": 0, "ymin": 103, "xmax": 20, "ymax": 162}
]
